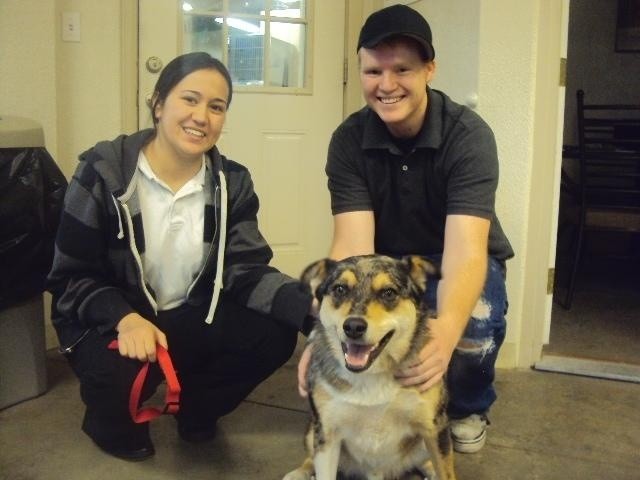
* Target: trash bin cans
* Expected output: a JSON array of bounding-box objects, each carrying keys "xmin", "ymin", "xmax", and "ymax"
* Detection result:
[{"xmin": 0, "ymin": 116, "xmax": 50, "ymax": 411}]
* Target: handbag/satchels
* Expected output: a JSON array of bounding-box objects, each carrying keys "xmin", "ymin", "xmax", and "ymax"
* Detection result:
[{"xmin": 0, "ymin": 147, "xmax": 69, "ymax": 311}]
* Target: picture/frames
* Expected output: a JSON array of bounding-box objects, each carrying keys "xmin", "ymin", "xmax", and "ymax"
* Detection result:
[{"xmin": 613, "ymin": 0, "xmax": 640, "ymax": 53}]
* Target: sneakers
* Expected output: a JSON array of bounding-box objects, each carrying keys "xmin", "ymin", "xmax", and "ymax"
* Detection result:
[{"xmin": 451, "ymin": 414, "xmax": 486, "ymax": 452}]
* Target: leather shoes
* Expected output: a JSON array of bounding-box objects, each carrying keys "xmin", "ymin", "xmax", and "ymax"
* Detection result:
[
  {"xmin": 102, "ymin": 436, "xmax": 156, "ymax": 461},
  {"xmin": 173, "ymin": 413, "xmax": 216, "ymax": 441}
]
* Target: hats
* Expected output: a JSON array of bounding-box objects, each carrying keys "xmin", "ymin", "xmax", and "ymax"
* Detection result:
[{"xmin": 357, "ymin": 3, "xmax": 435, "ymax": 62}]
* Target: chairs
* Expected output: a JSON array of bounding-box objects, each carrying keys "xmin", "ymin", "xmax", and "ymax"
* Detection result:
[{"xmin": 561, "ymin": 88, "xmax": 640, "ymax": 311}]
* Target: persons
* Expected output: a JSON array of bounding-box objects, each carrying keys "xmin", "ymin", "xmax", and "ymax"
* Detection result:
[
  {"xmin": 45, "ymin": 51, "xmax": 320, "ymax": 460},
  {"xmin": 297, "ymin": 3, "xmax": 515, "ymax": 454}
]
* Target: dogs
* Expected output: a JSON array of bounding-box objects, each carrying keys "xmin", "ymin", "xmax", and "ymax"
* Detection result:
[{"xmin": 282, "ymin": 254, "xmax": 456, "ymax": 480}]
